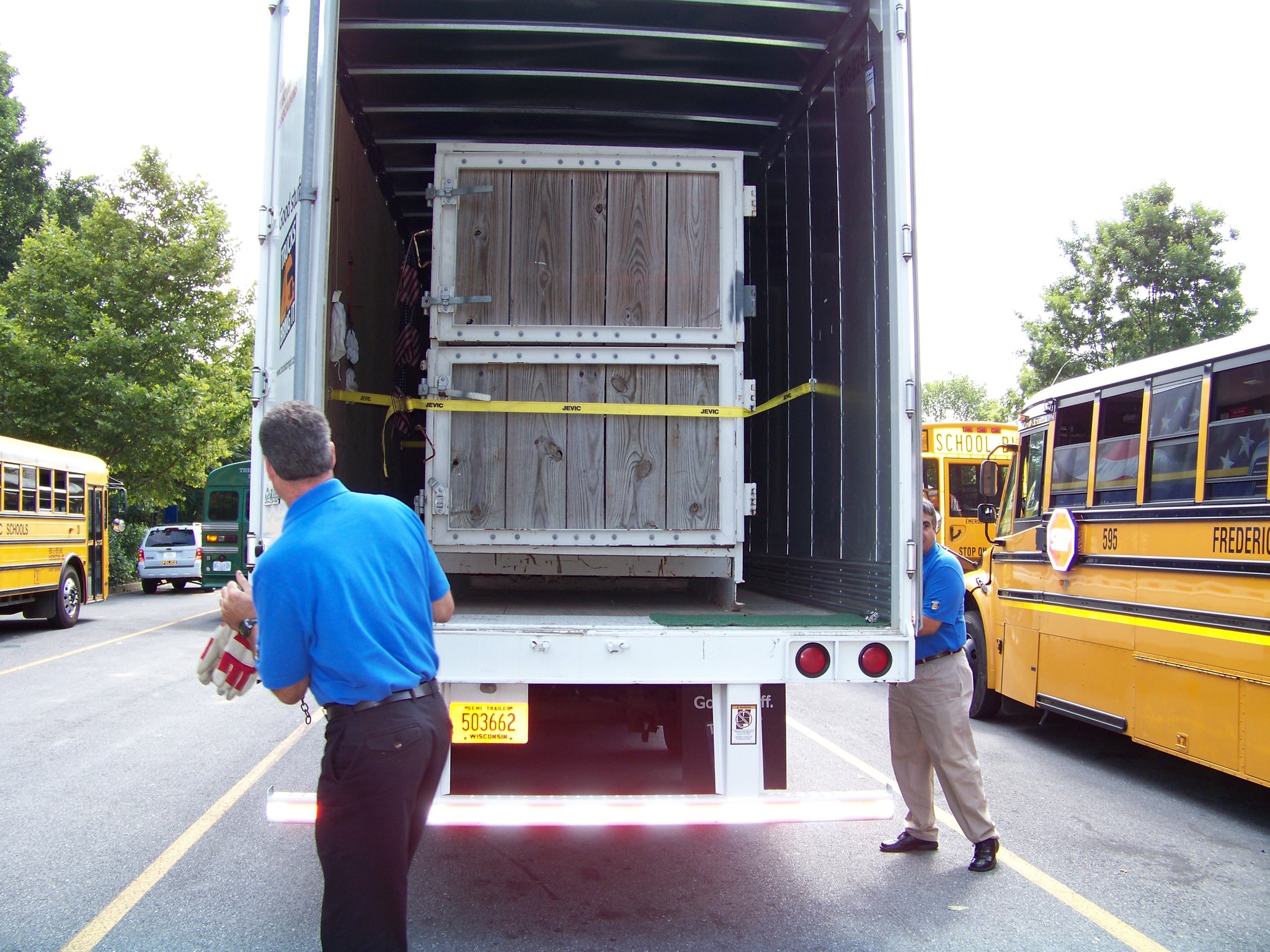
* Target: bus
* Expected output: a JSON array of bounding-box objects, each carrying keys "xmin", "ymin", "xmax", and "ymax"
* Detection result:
[
  {"xmin": 920, "ymin": 422, "xmax": 1026, "ymax": 576},
  {"xmin": 200, "ymin": 460, "xmax": 248, "ymax": 587},
  {"xmin": 960, "ymin": 319, "xmax": 1269, "ymax": 790},
  {"xmin": 0, "ymin": 434, "xmax": 128, "ymax": 630}
]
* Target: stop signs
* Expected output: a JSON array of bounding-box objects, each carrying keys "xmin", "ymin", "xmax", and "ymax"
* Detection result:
[{"xmin": 1046, "ymin": 507, "xmax": 1079, "ymax": 572}]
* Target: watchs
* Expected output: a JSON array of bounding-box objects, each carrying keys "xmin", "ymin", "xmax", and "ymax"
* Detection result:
[{"xmin": 239, "ymin": 618, "xmax": 257, "ymax": 637}]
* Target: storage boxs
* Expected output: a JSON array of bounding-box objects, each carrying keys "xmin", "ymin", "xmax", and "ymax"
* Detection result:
[{"xmin": 414, "ymin": 138, "xmax": 758, "ymax": 608}]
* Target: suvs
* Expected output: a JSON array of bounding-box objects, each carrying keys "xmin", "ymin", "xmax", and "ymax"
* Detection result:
[{"xmin": 136, "ymin": 522, "xmax": 216, "ymax": 594}]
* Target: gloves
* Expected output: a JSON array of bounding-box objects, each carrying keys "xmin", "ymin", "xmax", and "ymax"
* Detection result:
[{"xmin": 195, "ymin": 622, "xmax": 257, "ymax": 701}]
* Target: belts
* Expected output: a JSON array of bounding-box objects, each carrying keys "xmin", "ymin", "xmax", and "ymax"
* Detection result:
[
  {"xmin": 915, "ymin": 647, "xmax": 962, "ymax": 666},
  {"xmin": 326, "ymin": 681, "xmax": 440, "ymax": 721}
]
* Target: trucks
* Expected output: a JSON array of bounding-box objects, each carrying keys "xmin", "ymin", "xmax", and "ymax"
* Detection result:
[{"xmin": 244, "ymin": 2, "xmax": 928, "ymax": 830}]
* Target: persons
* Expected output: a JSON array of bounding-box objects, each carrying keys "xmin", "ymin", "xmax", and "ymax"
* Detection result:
[
  {"xmin": 217, "ymin": 401, "xmax": 457, "ymax": 952},
  {"xmin": 879, "ymin": 496, "xmax": 1001, "ymax": 872}
]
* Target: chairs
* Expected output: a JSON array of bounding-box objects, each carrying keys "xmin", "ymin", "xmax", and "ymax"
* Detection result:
[{"xmin": 923, "ymin": 489, "xmax": 940, "ymax": 512}]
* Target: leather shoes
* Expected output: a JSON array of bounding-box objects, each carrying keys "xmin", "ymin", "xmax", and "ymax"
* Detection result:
[
  {"xmin": 880, "ymin": 832, "xmax": 939, "ymax": 853},
  {"xmin": 968, "ymin": 834, "xmax": 999, "ymax": 871}
]
